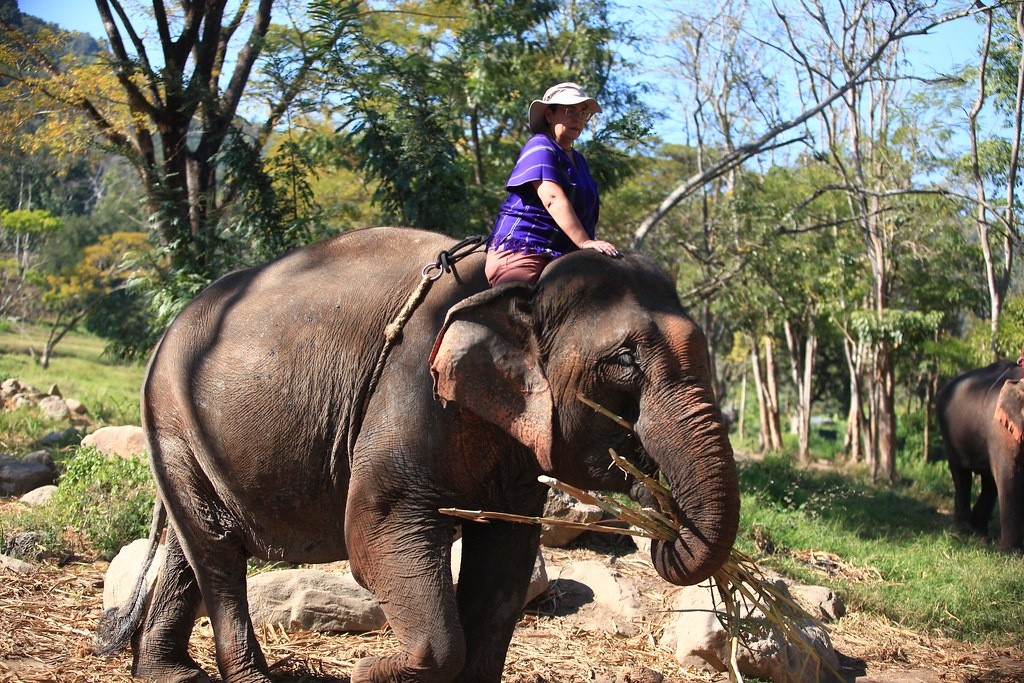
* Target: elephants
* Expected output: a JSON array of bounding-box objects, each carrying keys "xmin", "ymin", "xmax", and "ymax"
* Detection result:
[
  {"xmin": 92, "ymin": 224, "xmax": 744, "ymax": 683},
  {"xmin": 936, "ymin": 359, "xmax": 1024, "ymax": 559}
]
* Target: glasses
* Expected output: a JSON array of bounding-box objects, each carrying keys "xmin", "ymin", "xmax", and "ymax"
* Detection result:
[{"xmin": 565, "ymin": 108, "xmax": 591, "ymax": 121}]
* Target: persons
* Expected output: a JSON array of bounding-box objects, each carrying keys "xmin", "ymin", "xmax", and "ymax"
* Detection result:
[{"xmin": 485, "ymin": 82, "xmax": 619, "ymax": 288}]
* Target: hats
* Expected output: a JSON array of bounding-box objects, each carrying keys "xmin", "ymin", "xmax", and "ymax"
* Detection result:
[{"xmin": 528, "ymin": 82, "xmax": 603, "ymax": 135}]
